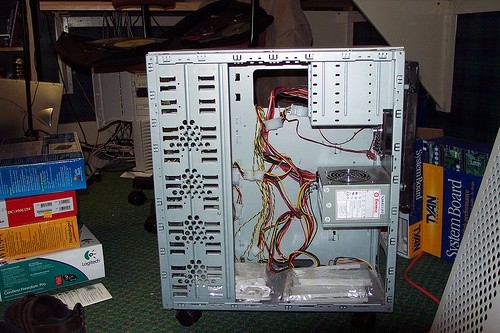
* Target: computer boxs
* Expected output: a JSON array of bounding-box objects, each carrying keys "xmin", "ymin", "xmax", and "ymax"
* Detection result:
[{"xmin": 148, "ymin": 46, "xmax": 404, "ymax": 315}]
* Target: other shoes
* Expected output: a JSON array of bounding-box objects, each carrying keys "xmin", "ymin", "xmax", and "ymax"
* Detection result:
[{"xmin": 4, "ymin": 293, "xmax": 85, "ymax": 333}]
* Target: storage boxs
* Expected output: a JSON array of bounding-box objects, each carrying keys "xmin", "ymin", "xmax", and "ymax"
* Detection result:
[{"xmin": 0, "ymin": 132, "xmax": 106, "ymax": 305}]
